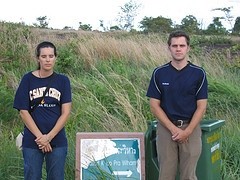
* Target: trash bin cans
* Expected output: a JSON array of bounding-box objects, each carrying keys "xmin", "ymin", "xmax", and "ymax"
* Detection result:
[{"xmin": 144, "ymin": 118, "xmax": 226, "ymax": 180}]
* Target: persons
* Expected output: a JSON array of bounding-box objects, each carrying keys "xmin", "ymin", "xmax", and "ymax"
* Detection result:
[
  {"xmin": 147, "ymin": 30, "xmax": 208, "ymax": 180},
  {"xmin": 13, "ymin": 41, "xmax": 72, "ymax": 180}
]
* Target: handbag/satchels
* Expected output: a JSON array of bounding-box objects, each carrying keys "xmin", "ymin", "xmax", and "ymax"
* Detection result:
[{"xmin": 15, "ymin": 132, "xmax": 25, "ymax": 151}]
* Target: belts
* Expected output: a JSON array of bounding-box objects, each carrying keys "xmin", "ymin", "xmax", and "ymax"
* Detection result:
[{"xmin": 172, "ymin": 121, "xmax": 189, "ymax": 126}]
